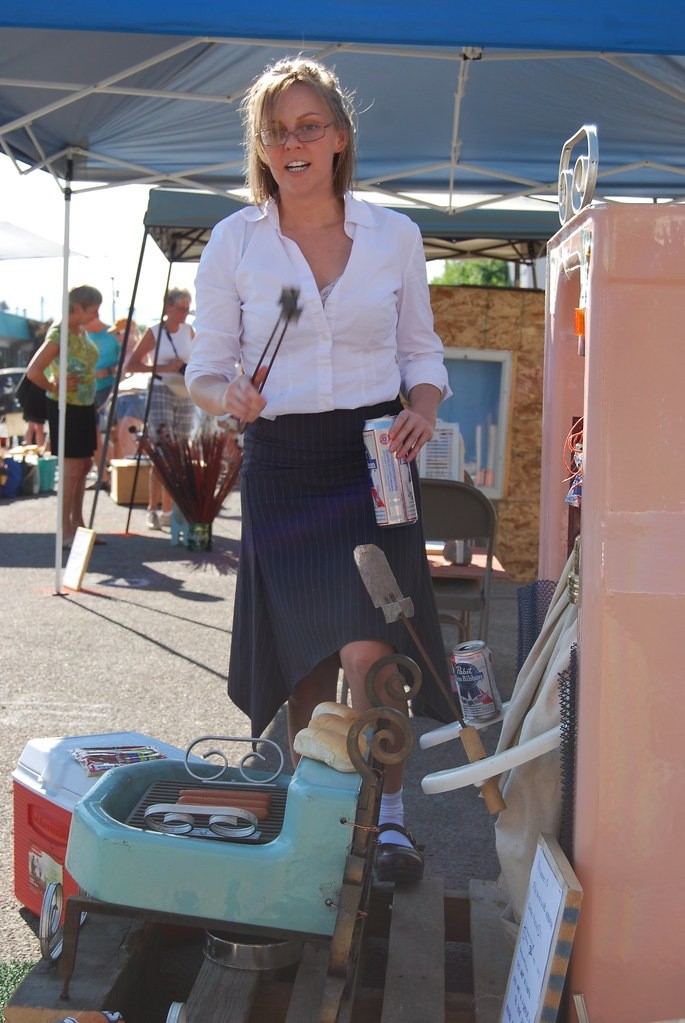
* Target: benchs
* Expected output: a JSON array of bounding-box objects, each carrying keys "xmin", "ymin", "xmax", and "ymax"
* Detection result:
[{"xmin": 109, "ymin": 457, "xmax": 206, "ymax": 504}]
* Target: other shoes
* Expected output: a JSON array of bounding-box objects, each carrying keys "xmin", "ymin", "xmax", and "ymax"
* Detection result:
[
  {"xmin": 94, "ymin": 539, "xmax": 106, "ymax": 545},
  {"xmin": 145, "ymin": 510, "xmax": 161, "ymax": 529},
  {"xmin": 373, "ymin": 823, "xmax": 426, "ymax": 884},
  {"xmin": 160, "ymin": 514, "xmax": 171, "ymax": 525},
  {"xmin": 85, "ymin": 481, "xmax": 109, "ymax": 490},
  {"xmin": 62, "ymin": 543, "xmax": 71, "ymax": 550}
]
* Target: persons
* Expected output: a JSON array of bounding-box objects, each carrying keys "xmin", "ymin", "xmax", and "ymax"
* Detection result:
[
  {"xmin": 14, "ymin": 283, "xmax": 197, "ymax": 553},
  {"xmin": 181, "ymin": 54, "xmax": 455, "ymax": 882}
]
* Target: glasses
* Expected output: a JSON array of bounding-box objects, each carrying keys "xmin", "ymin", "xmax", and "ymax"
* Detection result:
[{"xmin": 255, "ymin": 119, "xmax": 334, "ymax": 148}]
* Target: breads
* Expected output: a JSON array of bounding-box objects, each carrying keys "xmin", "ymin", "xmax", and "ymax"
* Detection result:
[{"xmin": 293, "ymin": 701, "xmax": 369, "ymax": 773}]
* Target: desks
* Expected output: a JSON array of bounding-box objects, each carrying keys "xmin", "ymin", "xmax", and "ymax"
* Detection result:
[{"xmin": 425, "ymin": 545, "xmax": 505, "ymax": 643}]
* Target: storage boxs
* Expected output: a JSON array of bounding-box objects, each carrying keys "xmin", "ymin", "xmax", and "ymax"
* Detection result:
[
  {"xmin": 39, "ymin": 454, "xmax": 59, "ymax": 493},
  {"xmin": 11, "ymin": 731, "xmax": 212, "ymax": 939}
]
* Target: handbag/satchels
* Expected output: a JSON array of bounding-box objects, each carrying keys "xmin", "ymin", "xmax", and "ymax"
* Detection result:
[
  {"xmin": 162, "ymin": 377, "xmax": 190, "ymax": 399},
  {"xmin": 22, "ymin": 454, "xmax": 40, "ymax": 494},
  {"xmin": 0, "ymin": 456, "xmax": 22, "ymax": 497}
]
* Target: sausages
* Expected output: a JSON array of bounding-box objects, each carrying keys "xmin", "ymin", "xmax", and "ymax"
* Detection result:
[{"xmin": 176, "ymin": 789, "xmax": 271, "ymax": 820}]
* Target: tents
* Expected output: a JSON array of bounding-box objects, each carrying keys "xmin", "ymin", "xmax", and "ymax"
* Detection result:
[
  {"xmin": 0, "ymin": 0, "xmax": 685, "ymax": 596},
  {"xmin": 84, "ymin": 186, "xmax": 561, "ymax": 531}
]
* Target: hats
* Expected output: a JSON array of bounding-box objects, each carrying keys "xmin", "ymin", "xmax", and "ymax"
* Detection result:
[
  {"xmin": 108, "ymin": 318, "xmax": 136, "ymax": 332},
  {"xmin": 86, "ymin": 318, "xmax": 110, "ymax": 333}
]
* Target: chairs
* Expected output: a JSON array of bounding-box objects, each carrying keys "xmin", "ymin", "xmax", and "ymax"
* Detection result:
[{"xmin": 416, "ymin": 469, "xmax": 498, "ymax": 646}]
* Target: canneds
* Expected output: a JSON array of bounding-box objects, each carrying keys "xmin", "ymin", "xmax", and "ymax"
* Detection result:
[
  {"xmin": 452, "ymin": 640, "xmax": 504, "ymax": 724},
  {"xmin": 363, "ymin": 416, "xmax": 419, "ymax": 528}
]
